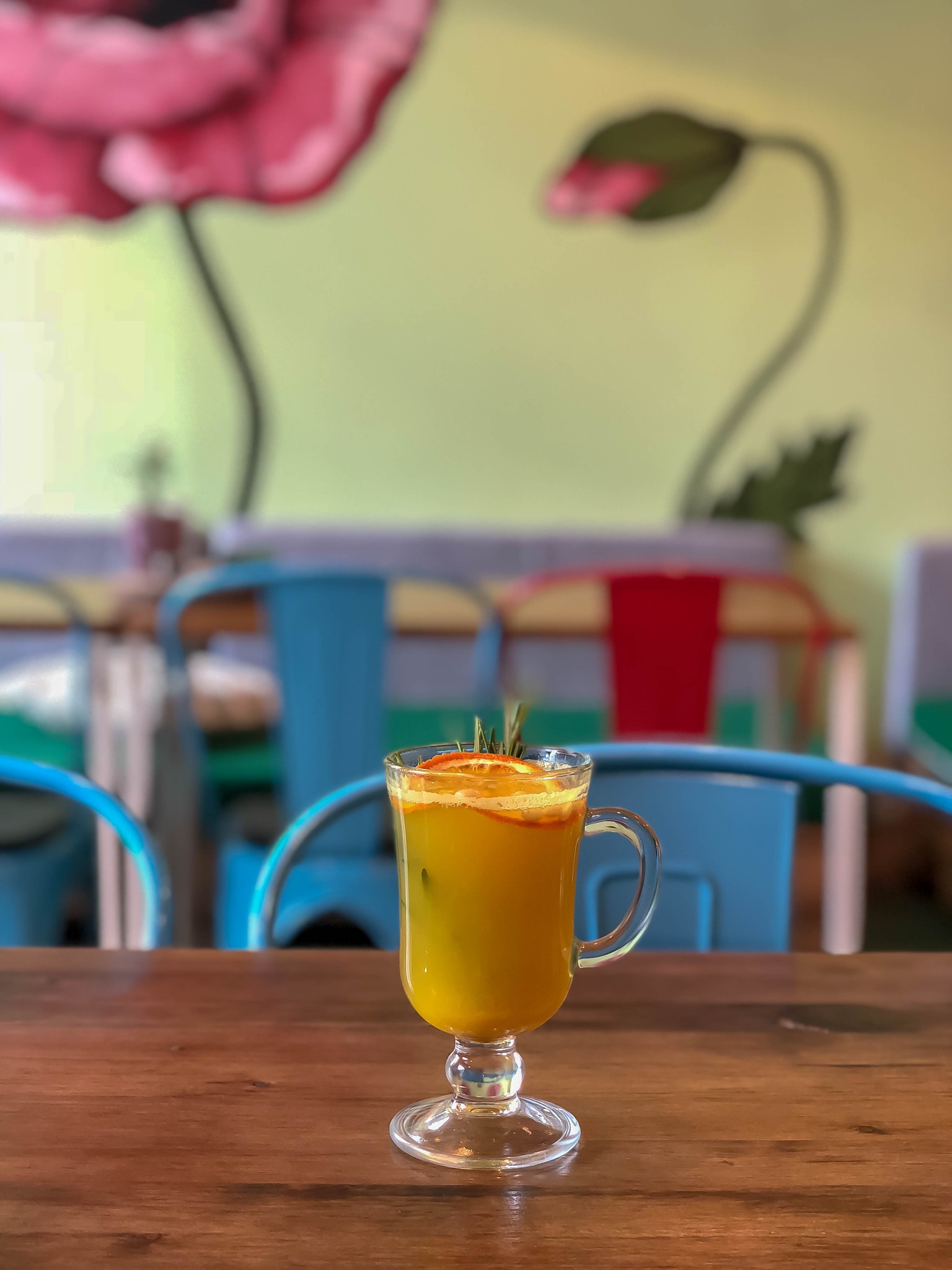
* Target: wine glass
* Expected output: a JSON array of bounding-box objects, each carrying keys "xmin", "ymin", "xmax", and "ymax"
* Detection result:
[{"xmin": 385, "ymin": 744, "xmax": 664, "ymax": 1170}]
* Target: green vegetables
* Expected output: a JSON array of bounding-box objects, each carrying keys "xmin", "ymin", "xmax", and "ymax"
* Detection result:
[{"xmin": 396, "ymin": 701, "xmax": 529, "ymax": 767}]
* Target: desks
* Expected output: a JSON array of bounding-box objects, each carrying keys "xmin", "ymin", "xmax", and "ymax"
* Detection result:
[
  {"xmin": 120, "ymin": 566, "xmax": 868, "ymax": 954},
  {"xmin": 0, "ymin": 945, "xmax": 952, "ymax": 1270},
  {"xmin": 0, "ymin": 571, "xmax": 123, "ymax": 949}
]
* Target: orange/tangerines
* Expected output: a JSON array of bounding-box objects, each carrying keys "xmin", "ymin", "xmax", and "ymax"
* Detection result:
[{"xmin": 395, "ymin": 753, "xmax": 564, "ymax": 813}]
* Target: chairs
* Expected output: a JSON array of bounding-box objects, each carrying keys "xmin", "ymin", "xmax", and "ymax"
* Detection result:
[
  {"xmin": 496, "ymin": 564, "xmax": 831, "ymax": 757},
  {"xmin": 155, "ymin": 556, "xmax": 503, "ymax": 951},
  {"xmin": 0, "ymin": 570, "xmax": 103, "ymax": 946},
  {"xmin": 0, "ymin": 758, "xmax": 172, "ymax": 948},
  {"xmin": 246, "ymin": 740, "xmax": 952, "ymax": 950}
]
